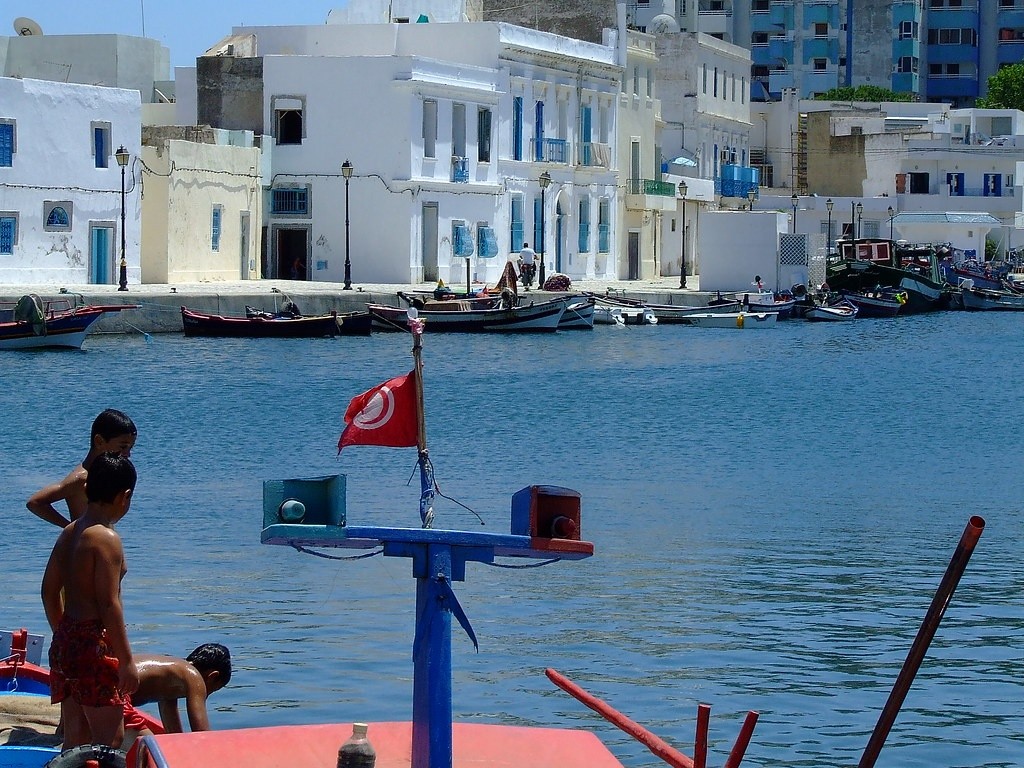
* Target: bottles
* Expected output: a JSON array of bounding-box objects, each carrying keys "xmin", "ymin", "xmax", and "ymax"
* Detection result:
[
  {"xmin": 478, "ymin": 289, "xmax": 483, "ymax": 298},
  {"xmin": 337, "ymin": 723, "xmax": 377, "ymax": 768}
]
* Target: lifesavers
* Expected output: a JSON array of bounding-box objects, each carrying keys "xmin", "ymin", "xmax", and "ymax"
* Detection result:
[
  {"xmin": 44, "ymin": 743, "xmax": 128, "ymax": 768},
  {"xmin": 791, "ymin": 283, "xmax": 806, "ymax": 293}
]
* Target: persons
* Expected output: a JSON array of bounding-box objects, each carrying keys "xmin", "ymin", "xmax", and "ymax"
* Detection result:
[
  {"xmin": 41, "ymin": 450, "xmax": 139, "ymax": 768},
  {"xmin": 517, "ymin": 243, "xmax": 539, "ymax": 278},
  {"xmin": 26, "ymin": 408, "xmax": 232, "ymax": 749}
]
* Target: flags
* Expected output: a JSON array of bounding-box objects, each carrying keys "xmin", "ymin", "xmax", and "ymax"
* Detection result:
[{"xmin": 337, "ymin": 368, "xmax": 418, "ymax": 456}]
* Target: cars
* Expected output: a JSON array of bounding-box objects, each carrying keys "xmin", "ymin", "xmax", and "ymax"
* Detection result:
[{"xmin": 825, "ymin": 240, "xmax": 839, "ymax": 256}]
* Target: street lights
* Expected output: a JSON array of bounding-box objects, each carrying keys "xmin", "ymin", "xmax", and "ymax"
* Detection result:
[
  {"xmin": 747, "ymin": 187, "xmax": 755, "ymax": 210},
  {"xmin": 857, "ymin": 201, "xmax": 863, "ymax": 238},
  {"xmin": 678, "ymin": 180, "xmax": 688, "ymax": 288},
  {"xmin": 888, "ymin": 206, "xmax": 894, "ymax": 239},
  {"xmin": 791, "ymin": 193, "xmax": 799, "ymax": 234},
  {"xmin": 825, "ymin": 199, "xmax": 834, "ymax": 264},
  {"xmin": 341, "ymin": 159, "xmax": 354, "ymax": 290},
  {"xmin": 538, "ymin": 170, "xmax": 551, "ymax": 288},
  {"xmin": 115, "ymin": 144, "xmax": 132, "ymax": 291}
]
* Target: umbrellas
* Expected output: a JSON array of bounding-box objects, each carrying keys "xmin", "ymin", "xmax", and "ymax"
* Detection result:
[{"xmin": 664, "ymin": 156, "xmax": 697, "ymax": 177}]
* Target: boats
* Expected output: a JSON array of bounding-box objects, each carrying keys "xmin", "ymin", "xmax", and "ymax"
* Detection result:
[
  {"xmin": 367, "ymin": 296, "xmax": 575, "ymax": 333},
  {"xmin": 682, "ymin": 312, "xmax": 778, "ymax": 328},
  {"xmin": 181, "ymin": 306, "xmax": 338, "ymax": 337},
  {"xmin": 0, "ymin": 476, "xmax": 761, "ymax": 768},
  {"xmin": 398, "ymin": 292, "xmax": 596, "ymax": 327},
  {"xmin": 827, "ymin": 235, "xmax": 1024, "ymax": 310},
  {"xmin": 245, "ymin": 305, "xmax": 374, "ymax": 337},
  {"xmin": 823, "ymin": 288, "xmax": 906, "ymax": 318},
  {"xmin": 582, "ymin": 291, "xmax": 740, "ymax": 325},
  {"xmin": 708, "ymin": 290, "xmax": 795, "ymax": 319},
  {"xmin": 0, "ymin": 294, "xmax": 142, "ymax": 353},
  {"xmin": 804, "ymin": 293, "xmax": 860, "ymax": 321}
]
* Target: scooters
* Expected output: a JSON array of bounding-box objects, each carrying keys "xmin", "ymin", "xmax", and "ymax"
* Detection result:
[{"xmin": 521, "ymin": 257, "xmax": 537, "ymax": 290}]
